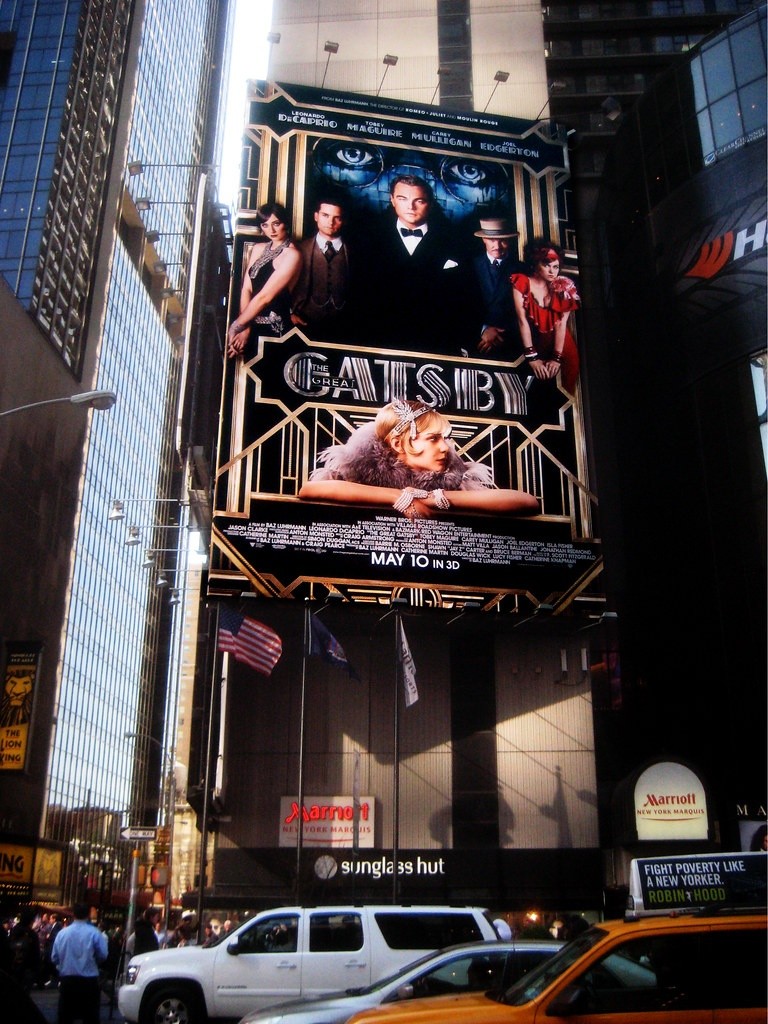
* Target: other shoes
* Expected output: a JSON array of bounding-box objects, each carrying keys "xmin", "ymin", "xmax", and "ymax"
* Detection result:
[{"xmin": 102, "ymin": 997, "xmax": 118, "ymax": 1008}]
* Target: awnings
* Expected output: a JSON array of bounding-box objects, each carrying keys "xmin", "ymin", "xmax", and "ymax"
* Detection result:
[{"xmin": 179, "ymin": 847, "xmax": 614, "ymax": 912}]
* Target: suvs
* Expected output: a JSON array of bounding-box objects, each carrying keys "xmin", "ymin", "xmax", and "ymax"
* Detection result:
[{"xmin": 117, "ymin": 902, "xmax": 503, "ymax": 1024}]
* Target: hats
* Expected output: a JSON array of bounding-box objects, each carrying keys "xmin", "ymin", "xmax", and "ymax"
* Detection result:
[
  {"xmin": 181, "ymin": 909, "xmax": 197, "ymax": 919},
  {"xmin": 474, "ymin": 218, "xmax": 520, "ymax": 238}
]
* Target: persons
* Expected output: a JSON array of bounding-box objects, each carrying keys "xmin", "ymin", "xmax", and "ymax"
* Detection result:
[
  {"xmin": 549, "ymin": 920, "xmax": 564, "ymax": 938},
  {"xmin": 225, "ymin": 203, "xmax": 302, "ymax": 364},
  {"xmin": 0, "ymin": 897, "xmax": 351, "ymax": 1024},
  {"xmin": 750, "ymin": 824, "xmax": 768, "ymax": 852},
  {"xmin": 508, "ymin": 237, "xmax": 578, "ymax": 396},
  {"xmin": 289, "ymin": 137, "xmax": 529, "ymax": 362},
  {"xmin": 465, "ymin": 918, "xmax": 601, "ymax": 993},
  {"xmin": 297, "ymin": 394, "xmax": 540, "ymax": 517}
]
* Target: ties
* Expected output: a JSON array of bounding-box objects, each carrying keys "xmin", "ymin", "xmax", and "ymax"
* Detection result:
[
  {"xmin": 325, "ymin": 241, "xmax": 335, "ymax": 263},
  {"xmin": 492, "ymin": 259, "xmax": 498, "ymax": 267}
]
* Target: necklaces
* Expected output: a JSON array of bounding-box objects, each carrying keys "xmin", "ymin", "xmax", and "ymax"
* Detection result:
[{"xmin": 250, "ymin": 238, "xmax": 291, "ymax": 279}]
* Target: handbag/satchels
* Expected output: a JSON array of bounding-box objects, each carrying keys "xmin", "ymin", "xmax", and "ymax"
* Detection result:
[{"xmin": 162, "ymin": 926, "xmax": 184, "ymax": 948}]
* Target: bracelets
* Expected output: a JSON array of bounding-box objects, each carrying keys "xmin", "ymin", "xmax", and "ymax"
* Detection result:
[
  {"xmin": 524, "ymin": 346, "xmax": 539, "ymax": 361},
  {"xmin": 432, "ymin": 488, "xmax": 449, "ymax": 510},
  {"xmin": 549, "ymin": 353, "xmax": 562, "ymax": 363},
  {"xmin": 231, "ymin": 321, "xmax": 244, "ymax": 333},
  {"xmin": 393, "ymin": 489, "xmax": 424, "ymax": 519}
]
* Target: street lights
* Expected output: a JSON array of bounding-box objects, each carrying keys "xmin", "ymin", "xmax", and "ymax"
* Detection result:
[
  {"xmin": 124, "ymin": 731, "xmax": 174, "ymax": 948},
  {"xmin": 73, "ymin": 808, "xmax": 133, "ymax": 918}
]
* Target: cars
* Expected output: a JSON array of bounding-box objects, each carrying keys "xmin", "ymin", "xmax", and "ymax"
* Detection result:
[
  {"xmin": 235, "ymin": 941, "xmax": 654, "ymax": 1024},
  {"xmin": 339, "ymin": 849, "xmax": 768, "ymax": 1024}
]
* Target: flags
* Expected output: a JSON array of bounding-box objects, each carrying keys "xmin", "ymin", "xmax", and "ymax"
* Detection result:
[
  {"xmin": 307, "ymin": 611, "xmax": 362, "ymax": 686},
  {"xmin": 218, "ymin": 606, "xmax": 281, "ymax": 675}
]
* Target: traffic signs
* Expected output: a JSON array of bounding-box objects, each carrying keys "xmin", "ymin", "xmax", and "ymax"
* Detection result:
[{"xmin": 118, "ymin": 827, "xmax": 157, "ymax": 841}]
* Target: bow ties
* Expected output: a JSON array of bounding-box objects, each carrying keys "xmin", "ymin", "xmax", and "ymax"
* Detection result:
[{"xmin": 401, "ymin": 228, "xmax": 424, "ymax": 239}]
